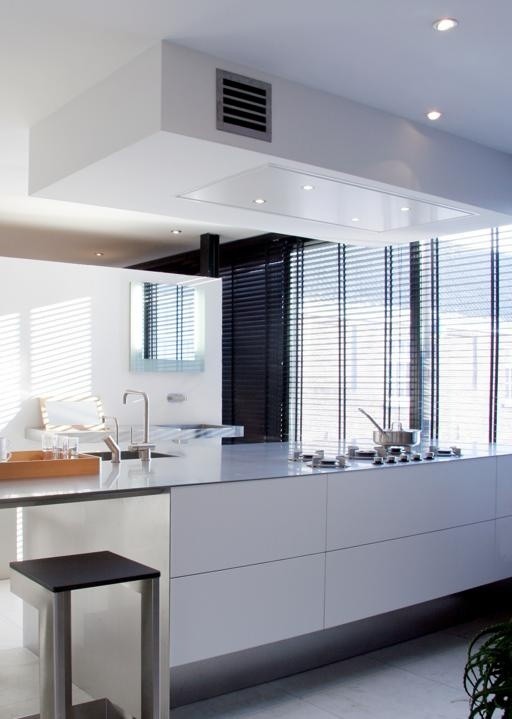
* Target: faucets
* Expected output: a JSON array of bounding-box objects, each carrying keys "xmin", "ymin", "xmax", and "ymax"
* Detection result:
[
  {"xmin": 103, "ymin": 416, "xmax": 120, "ymax": 450},
  {"xmin": 123, "ymin": 390, "xmax": 149, "ymax": 446}
]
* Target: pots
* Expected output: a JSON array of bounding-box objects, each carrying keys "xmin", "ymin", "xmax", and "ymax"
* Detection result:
[{"xmin": 371, "ymin": 421, "xmax": 422, "ymax": 447}]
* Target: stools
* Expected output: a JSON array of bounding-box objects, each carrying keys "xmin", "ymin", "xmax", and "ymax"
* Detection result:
[{"xmin": 9, "ymin": 551, "xmax": 164, "ymax": 717}]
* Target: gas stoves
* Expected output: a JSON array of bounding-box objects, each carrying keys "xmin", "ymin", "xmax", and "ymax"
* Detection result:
[{"xmin": 286, "ymin": 444, "xmax": 461, "ymax": 474}]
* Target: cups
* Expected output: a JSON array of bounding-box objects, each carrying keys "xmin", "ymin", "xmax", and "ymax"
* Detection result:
[
  {"xmin": 62, "ymin": 436, "xmax": 80, "ymax": 460},
  {"xmin": 0, "ymin": 436, "xmax": 10, "ymax": 463},
  {"xmin": 40, "ymin": 432, "xmax": 61, "ymax": 460}
]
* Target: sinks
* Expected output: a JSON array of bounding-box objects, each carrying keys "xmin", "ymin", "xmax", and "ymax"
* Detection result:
[{"xmin": 84, "ymin": 451, "xmax": 174, "ymax": 460}]
